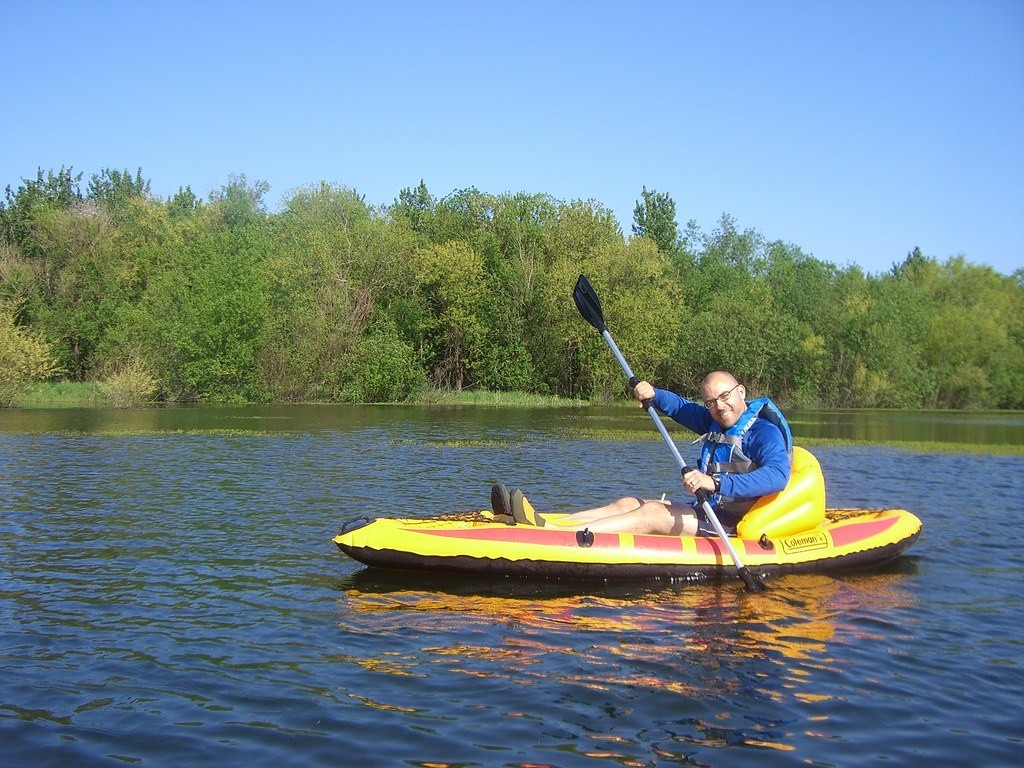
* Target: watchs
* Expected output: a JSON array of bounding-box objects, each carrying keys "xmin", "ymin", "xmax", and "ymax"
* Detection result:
[{"xmin": 712, "ymin": 474, "xmax": 720, "ymax": 494}]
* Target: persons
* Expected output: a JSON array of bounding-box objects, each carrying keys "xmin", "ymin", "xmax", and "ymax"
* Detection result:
[{"xmin": 491, "ymin": 371, "xmax": 791, "ymax": 537}]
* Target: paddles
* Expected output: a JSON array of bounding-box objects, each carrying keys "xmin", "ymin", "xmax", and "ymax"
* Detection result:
[{"xmin": 573, "ymin": 273, "xmax": 771, "ymax": 595}]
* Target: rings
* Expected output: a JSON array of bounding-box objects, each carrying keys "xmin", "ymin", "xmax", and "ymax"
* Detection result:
[{"xmin": 691, "ymin": 482, "xmax": 694, "ymax": 486}]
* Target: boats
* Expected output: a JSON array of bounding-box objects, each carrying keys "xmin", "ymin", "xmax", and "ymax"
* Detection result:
[{"xmin": 328, "ymin": 444, "xmax": 927, "ymax": 574}]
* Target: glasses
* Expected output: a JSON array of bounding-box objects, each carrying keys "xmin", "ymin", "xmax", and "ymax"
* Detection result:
[{"xmin": 704, "ymin": 384, "xmax": 739, "ymax": 409}]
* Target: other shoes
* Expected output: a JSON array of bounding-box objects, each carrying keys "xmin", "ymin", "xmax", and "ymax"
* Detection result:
[
  {"xmin": 491, "ymin": 484, "xmax": 513, "ymax": 516},
  {"xmin": 510, "ymin": 488, "xmax": 546, "ymax": 527}
]
